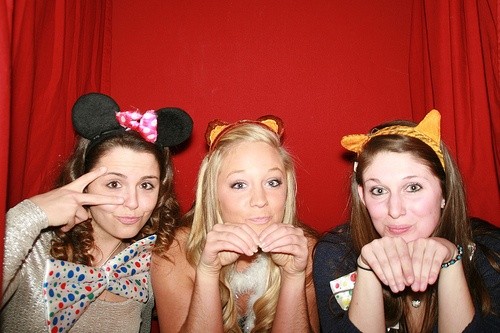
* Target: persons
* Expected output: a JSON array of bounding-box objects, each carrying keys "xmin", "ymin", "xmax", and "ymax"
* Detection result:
[
  {"xmin": 148, "ymin": 114, "xmax": 319, "ymax": 332},
  {"xmin": 311, "ymin": 110, "xmax": 500, "ymax": 333},
  {"xmin": 3, "ymin": 92, "xmax": 194, "ymax": 332}
]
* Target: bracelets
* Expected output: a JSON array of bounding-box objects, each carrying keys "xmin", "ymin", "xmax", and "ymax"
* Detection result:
[{"xmin": 441, "ymin": 243, "xmax": 464, "ymax": 267}]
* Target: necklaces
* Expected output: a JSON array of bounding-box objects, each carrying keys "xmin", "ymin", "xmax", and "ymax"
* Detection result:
[{"xmin": 408, "ymin": 291, "xmax": 426, "ymax": 308}]
